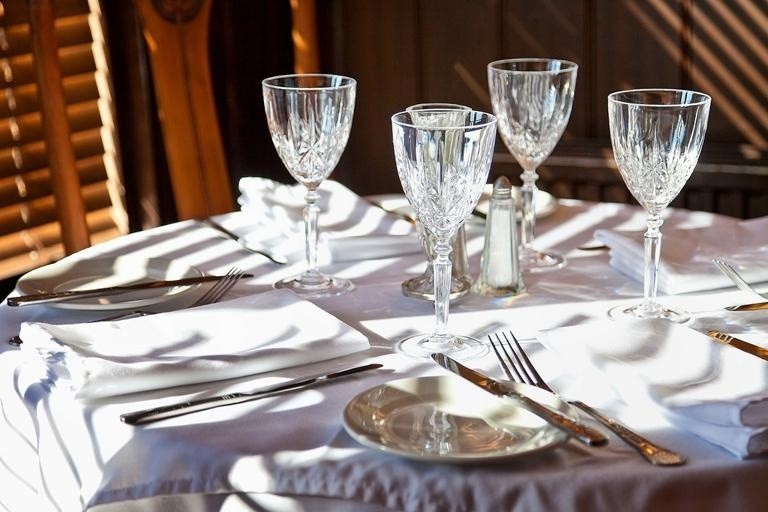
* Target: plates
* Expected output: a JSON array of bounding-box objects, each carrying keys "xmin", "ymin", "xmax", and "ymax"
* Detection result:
[
  {"xmin": 15, "ymin": 256, "xmax": 206, "ymax": 311},
  {"xmin": 341, "ymin": 376, "xmax": 580, "ymax": 465},
  {"xmin": 475, "ymin": 180, "xmax": 560, "ymax": 220}
]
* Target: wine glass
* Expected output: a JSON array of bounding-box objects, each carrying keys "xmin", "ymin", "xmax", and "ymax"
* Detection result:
[
  {"xmin": 261, "ymin": 73, "xmax": 359, "ymax": 297},
  {"xmin": 400, "ymin": 102, "xmax": 475, "ymax": 302},
  {"xmin": 479, "ymin": 59, "xmax": 580, "ymax": 275},
  {"xmin": 392, "ymin": 110, "xmax": 498, "ymax": 361},
  {"xmin": 607, "ymin": 88, "xmax": 712, "ymax": 325}
]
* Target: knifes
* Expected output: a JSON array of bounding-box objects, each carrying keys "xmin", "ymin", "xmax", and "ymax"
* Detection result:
[
  {"xmin": 8, "ymin": 274, "xmax": 255, "ymax": 308},
  {"xmin": 431, "ymin": 352, "xmax": 609, "ymax": 448},
  {"xmin": 120, "ymin": 361, "xmax": 384, "ymax": 425}
]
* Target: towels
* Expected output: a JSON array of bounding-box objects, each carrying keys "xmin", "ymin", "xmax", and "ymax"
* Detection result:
[
  {"xmin": 20, "ymin": 286, "xmax": 369, "ymax": 405},
  {"xmin": 537, "ymin": 316, "xmax": 768, "ymax": 462},
  {"xmin": 234, "ymin": 176, "xmax": 425, "ymax": 268},
  {"xmin": 593, "ymin": 225, "xmax": 768, "ymax": 299}
]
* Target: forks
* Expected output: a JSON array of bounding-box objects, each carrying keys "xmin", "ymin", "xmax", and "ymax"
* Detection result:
[
  {"xmin": 712, "ymin": 257, "xmax": 768, "ymax": 301},
  {"xmin": 488, "ymin": 330, "xmax": 688, "ymax": 467},
  {"xmin": 8, "ymin": 266, "xmax": 247, "ymax": 347}
]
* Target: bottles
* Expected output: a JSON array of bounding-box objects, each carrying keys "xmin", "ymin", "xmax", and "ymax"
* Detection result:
[{"xmin": 472, "ymin": 176, "xmax": 527, "ymax": 296}]
